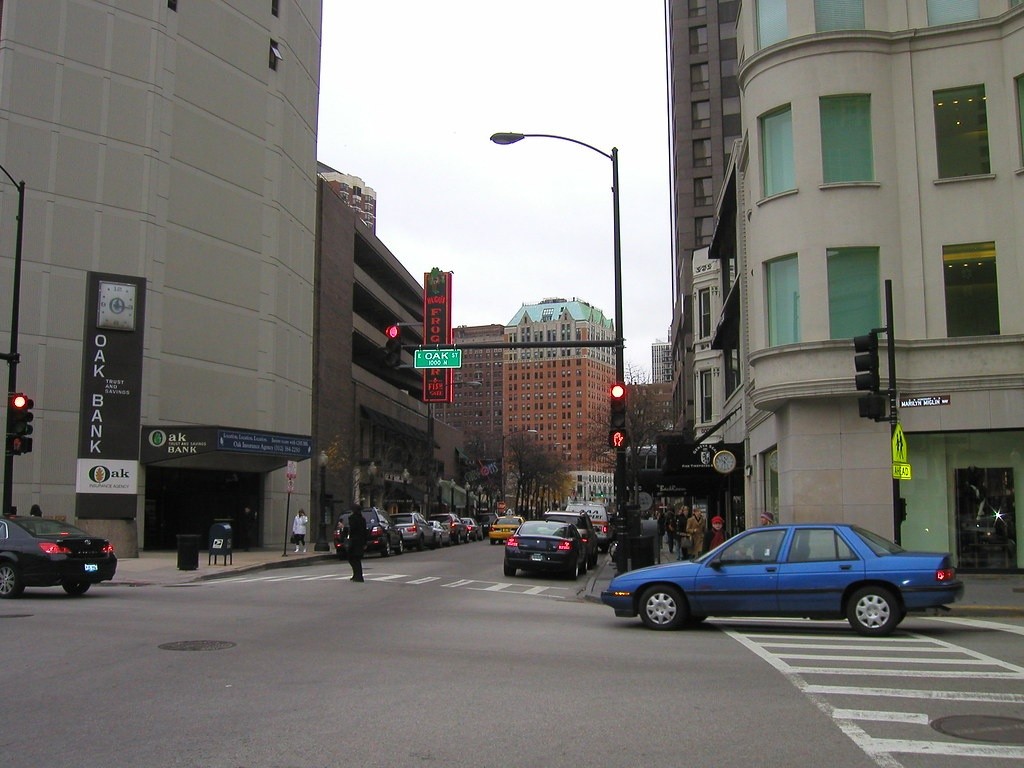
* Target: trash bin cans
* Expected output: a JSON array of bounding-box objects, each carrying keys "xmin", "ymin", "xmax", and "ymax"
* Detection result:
[
  {"xmin": 176, "ymin": 534, "xmax": 202, "ymax": 570},
  {"xmin": 641, "ymin": 520, "xmax": 658, "ymax": 558},
  {"xmin": 630, "ymin": 534, "xmax": 655, "ymax": 571}
]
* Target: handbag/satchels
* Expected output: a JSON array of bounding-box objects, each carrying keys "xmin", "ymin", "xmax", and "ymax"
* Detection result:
[
  {"xmin": 681, "ymin": 532, "xmax": 693, "ymax": 548},
  {"xmin": 290, "ymin": 534, "xmax": 298, "ymax": 543}
]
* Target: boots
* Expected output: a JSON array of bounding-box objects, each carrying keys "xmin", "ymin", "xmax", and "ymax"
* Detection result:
[
  {"xmin": 302, "ymin": 543, "xmax": 307, "ymax": 553},
  {"xmin": 294, "ymin": 545, "xmax": 299, "ymax": 552}
]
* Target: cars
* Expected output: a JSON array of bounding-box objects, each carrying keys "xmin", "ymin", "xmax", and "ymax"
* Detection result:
[
  {"xmin": 460, "ymin": 517, "xmax": 484, "ymax": 541},
  {"xmin": 426, "ymin": 520, "xmax": 452, "ymax": 548},
  {"xmin": 600, "ymin": 523, "xmax": 965, "ymax": 638},
  {"xmin": 488, "ymin": 508, "xmax": 524, "ymax": 545},
  {"xmin": 0, "ymin": 515, "xmax": 117, "ymax": 599},
  {"xmin": 503, "ymin": 520, "xmax": 589, "ymax": 582}
]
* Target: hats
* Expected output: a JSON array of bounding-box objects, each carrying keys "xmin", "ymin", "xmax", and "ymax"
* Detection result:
[
  {"xmin": 351, "ymin": 503, "xmax": 361, "ymax": 514},
  {"xmin": 760, "ymin": 512, "xmax": 773, "ymax": 523},
  {"xmin": 712, "ymin": 516, "xmax": 724, "ymax": 523}
]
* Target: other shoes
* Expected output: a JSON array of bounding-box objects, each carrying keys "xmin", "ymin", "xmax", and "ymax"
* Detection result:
[{"xmin": 350, "ymin": 577, "xmax": 363, "ymax": 582}]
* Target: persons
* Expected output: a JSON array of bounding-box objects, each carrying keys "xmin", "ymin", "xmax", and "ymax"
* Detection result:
[
  {"xmin": 337, "ymin": 518, "xmax": 348, "ymax": 543},
  {"xmin": 293, "ymin": 509, "xmax": 309, "ymax": 553},
  {"xmin": 759, "ymin": 511, "xmax": 774, "ymax": 526},
  {"xmin": 656, "ymin": 504, "xmax": 706, "ymax": 561},
  {"xmin": 347, "ymin": 503, "xmax": 368, "ymax": 583},
  {"xmin": 30, "ymin": 504, "xmax": 42, "ymax": 518},
  {"xmin": 701, "ymin": 515, "xmax": 732, "ymax": 555},
  {"xmin": 241, "ymin": 506, "xmax": 256, "ymax": 551}
]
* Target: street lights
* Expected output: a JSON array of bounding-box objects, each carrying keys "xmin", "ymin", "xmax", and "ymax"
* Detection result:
[
  {"xmin": 367, "ymin": 461, "xmax": 377, "ymax": 508},
  {"xmin": 501, "ymin": 429, "xmax": 538, "ymax": 502},
  {"xmin": 425, "ymin": 381, "xmax": 483, "ymax": 521},
  {"xmin": 477, "ymin": 484, "xmax": 483, "ymax": 520},
  {"xmin": 465, "ymin": 482, "xmax": 471, "ymax": 517},
  {"xmin": 450, "ymin": 479, "xmax": 456, "ymax": 514},
  {"xmin": 402, "ymin": 469, "xmax": 409, "ymax": 512},
  {"xmin": 486, "ymin": 130, "xmax": 632, "ymax": 577}
]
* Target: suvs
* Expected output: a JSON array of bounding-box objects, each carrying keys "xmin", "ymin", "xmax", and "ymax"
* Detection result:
[
  {"xmin": 430, "ymin": 512, "xmax": 470, "ymax": 545},
  {"xmin": 476, "ymin": 513, "xmax": 500, "ymax": 537},
  {"xmin": 334, "ymin": 506, "xmax": 404, "ymax": 561},
  {"xmin": 542, "ymin": 508, "xmax": 601, "ymax": 570},
  {"xmin": 388, "ymin": 511, "xmax": 436, "ymax": 552}
]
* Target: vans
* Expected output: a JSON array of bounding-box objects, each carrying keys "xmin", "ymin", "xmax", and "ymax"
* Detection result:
[{"xmin": 565, "ymin": 500, "xmax": 610, "ymax": 554}]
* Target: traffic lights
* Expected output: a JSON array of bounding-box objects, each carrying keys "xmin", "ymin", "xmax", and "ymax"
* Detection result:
[
  {"xmin": 11, "ymin": 436, "xmax": 27, "ymax": 454},
  {"xmin": 25, "ymin": 398, "xmax": 34, "ymax": 435},
  {"xmin": 385, "ymin": 324, "xmax": 402, "ymax": 367},
  {"xmin": 610, "ymin": 383, "xmax": 626, "ymax": 428},
  {"xmin": 609, "ymin": 429, "xmax": 626, "ymax": 449},
  {"xmin": 10, "ymin": 392, "xmax": 28, "ymax": 438},
  {"xmin": 853, "ymin": 332, "xmax": 880, "ymax": 392}
]
